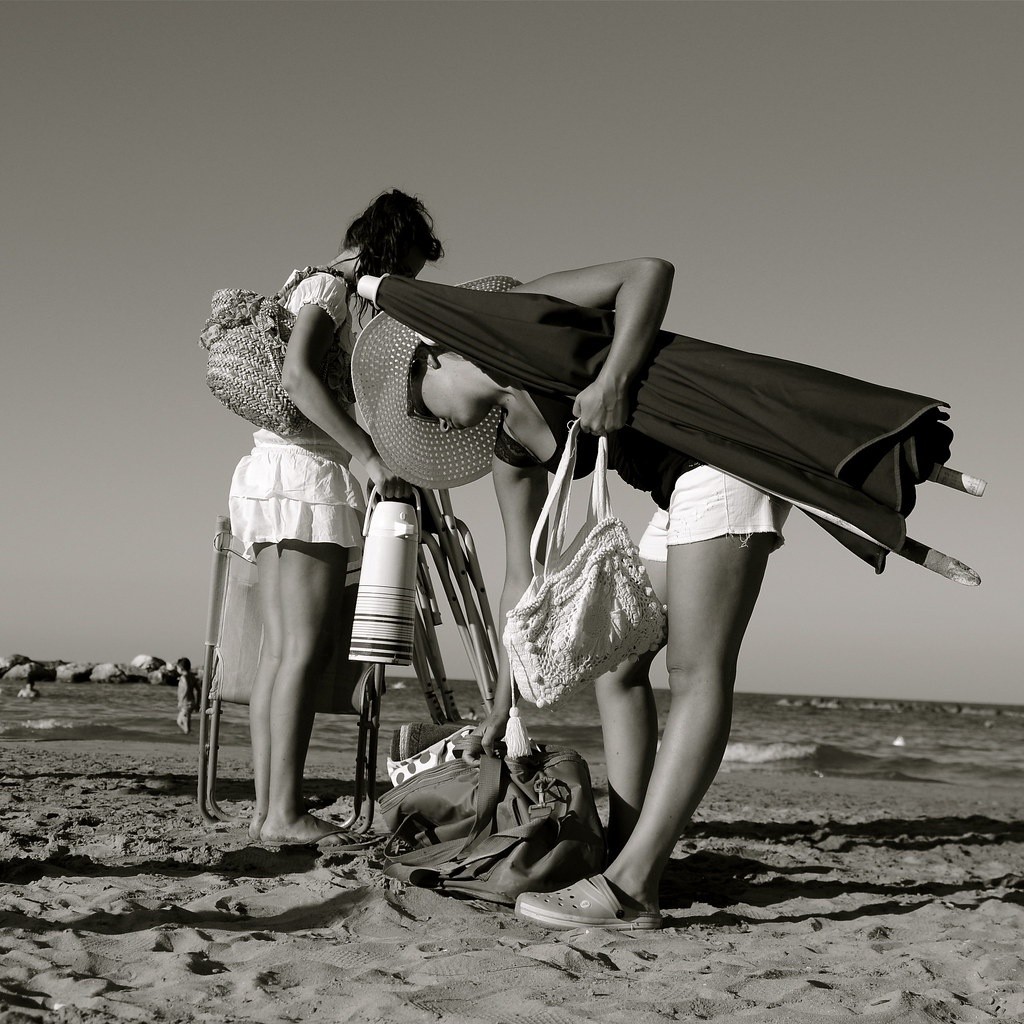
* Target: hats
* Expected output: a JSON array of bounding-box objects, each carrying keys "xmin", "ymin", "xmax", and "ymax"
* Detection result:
[{"xmin": 349, "ymin": 274, "xmax": 525, "ymax": 491}]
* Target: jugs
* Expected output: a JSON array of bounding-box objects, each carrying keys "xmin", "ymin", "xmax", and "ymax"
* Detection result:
[{"xmin": 348, "ymin": 472, "xmax": 430, "ymax": 667}]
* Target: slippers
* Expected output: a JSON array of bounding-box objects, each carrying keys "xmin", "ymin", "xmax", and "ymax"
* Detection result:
[
  {"xmin": 246, "ymin": 819, "xmax": 387, "ymax": 852},
  {"xmin": 514, "ymin": 873, "xmax": 663, "ymax": 929}
]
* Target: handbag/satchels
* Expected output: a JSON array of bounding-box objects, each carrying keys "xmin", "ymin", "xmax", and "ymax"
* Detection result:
[
  {"xmin": 501, "ymin": 515, "xmax": 670, "ymax": 713},
  {"xmin": 386, "ymin": 724, "xmax": 542, "ymax": 787},
  {"xmin": 376, "ymin": 724, "xmax": 610, "ymax": 904},
  {"xmin": 191, "ymin": 265, "xmax": 358, "ymax": 441}
]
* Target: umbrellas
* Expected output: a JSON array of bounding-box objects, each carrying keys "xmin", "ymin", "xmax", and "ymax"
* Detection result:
[{"xmin": 359, "ymin": 271, "xmax": 989, "ymax": 585}]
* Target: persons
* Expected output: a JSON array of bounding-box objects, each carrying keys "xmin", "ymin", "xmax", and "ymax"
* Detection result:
[
  {"xmin": 355, "ymin": 259, "xmax": 792, "ymax": 927},
  {"xmin": 17, "ymin": 680, "xmax": 42, "ymax": 699},
  {"xmin": 231, "ymin": 190, "xmax": 440, "ymax": 847},
  {"xmin": 176, "ymin": 657, "xmax": 199, "ymax": 735}
]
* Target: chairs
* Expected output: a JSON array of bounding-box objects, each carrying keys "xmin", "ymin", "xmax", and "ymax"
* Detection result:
[{"xmin": 196, "ymin": 515, "xmax": 389, "ymax": 839}]
